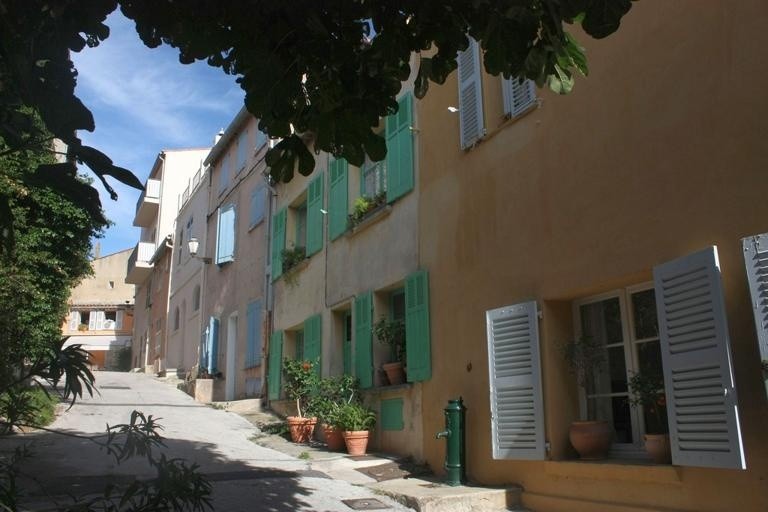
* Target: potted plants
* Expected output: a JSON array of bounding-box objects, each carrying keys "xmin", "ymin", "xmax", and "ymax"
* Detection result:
[
  {"xmin": 280, "ymin": 356, "xmax": 378, "ymax": 456},
  {"xmin": 373, "ymin": 314, "xmax": 405, "ymax": 385},
  {"xmin": 565, "ymin": 334, "xmax": 669, "ymax": 461}
]
